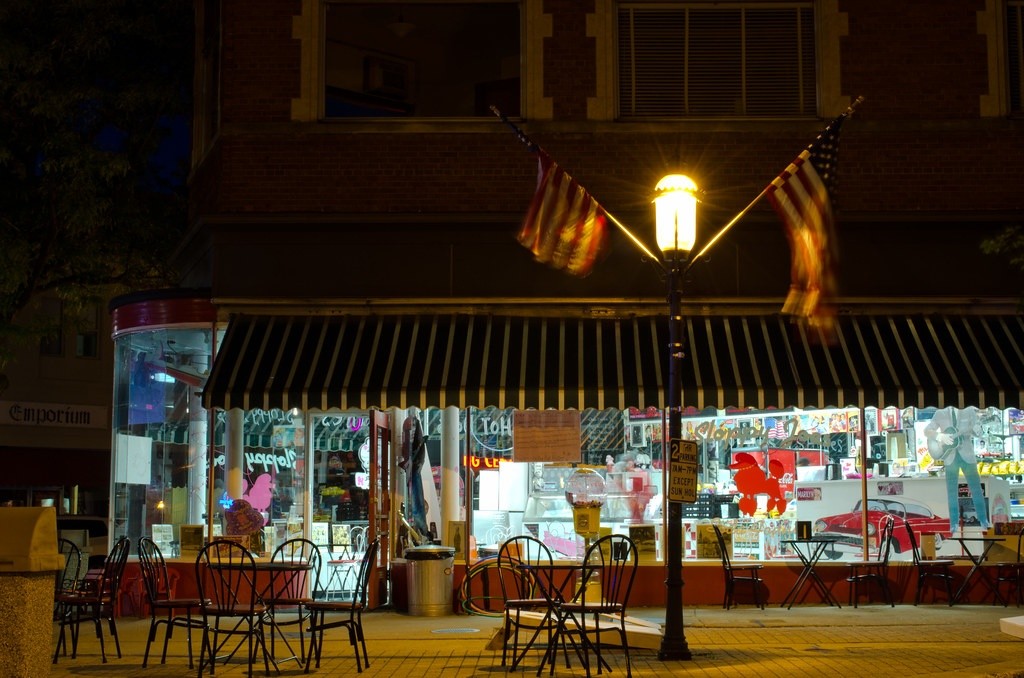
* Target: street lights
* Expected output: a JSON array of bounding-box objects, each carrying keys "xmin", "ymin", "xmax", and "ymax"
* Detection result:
[{"xmin": 651, "ymin": 172, "xmax": 699, "ymax": 662}]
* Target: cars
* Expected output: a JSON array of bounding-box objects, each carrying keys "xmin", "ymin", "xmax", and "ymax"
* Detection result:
[
  {"xmin": 816, "ymin": 498, "xmax": 953, "ymax": 562},
  {"xmin": 540, "ymin": 466, "xmax": 611, "ymax": 516}
]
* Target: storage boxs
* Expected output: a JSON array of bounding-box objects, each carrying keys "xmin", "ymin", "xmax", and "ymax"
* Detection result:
[{"xmin": 796, "ymin": 465, "xmax": 832, "ymax": 482}]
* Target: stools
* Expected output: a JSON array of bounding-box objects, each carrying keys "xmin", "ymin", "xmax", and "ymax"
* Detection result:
[{"xmin": 327, "ymin": 559, "xmax": 357, "ymax": 600}]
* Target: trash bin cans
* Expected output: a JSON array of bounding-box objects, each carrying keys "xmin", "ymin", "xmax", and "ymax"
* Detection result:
[
  {"xmin": 404, "ymin": 544, "xmax": 456, "ymax": 617},
  {"xmin": 58, "ymin": 530, "xmax": 92, "ymax": 585}
]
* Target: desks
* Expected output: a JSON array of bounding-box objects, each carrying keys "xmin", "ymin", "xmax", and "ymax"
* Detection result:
[
  {"xmin": 205, "ymin": 564, "xmax": 313, "ymax": 678},
  {"xmin": 780, "ymin": 540, "xmax": 841, "ymax": 609},
  {"xmin": 948, "ymin": 538, "xmax": 1005, "ymax": 607},
  {"xmin": 515, "ymin": 564, "xmax": 612, "ymax": 675}
]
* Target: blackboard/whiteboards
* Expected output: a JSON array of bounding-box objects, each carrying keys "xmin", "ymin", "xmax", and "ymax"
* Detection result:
[{"xmin": 585, "ymin": 417, "xmax": 614, "ymax": 466}]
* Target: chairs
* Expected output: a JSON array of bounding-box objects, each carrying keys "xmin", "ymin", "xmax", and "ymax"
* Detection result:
[
  {"xmin": 846, "ymin": 518, "xmax": 894, "ymax": 608},
  {"xmin": 550, "ymin": 535, "xmax": 638, "ymax": 678},
  {"xmin": 58, "ymin": 537, "xmax": 130, "ymax": 663},
  {"xmin": 262, "ymin": 539, "xmax": 322, "ymax": 663},
  {"xmin": 52, "ymin": 539, "xmax": 82, "ymax": 665},
  {"xmin": 138, "ymin": 564, "xmax": 179, "ymax": 621},
  {"xmin": 195, "ymin": 539, "xmax": 267, "ymax": 678},
  {"xmin": 713, "ymin": 524, "xmax": 763, "ymax": 610},
  {"xmin": 305, "ymin": 539, "xmax": 378, "ymax": 673},
  {"xmin": 906, "ymin": 521, "xmax": 956, "ymax": 606},
  {"xmin": 137, "ymin": 536, "xmax": 210, "ymax": 668},
  {"xmin": 498, "ymin": 535, "xmax": 571, "ymax": 670},
  {"xmin": 996, "ymin": 529, "xmax": 1023, "ymax": 608}
]
